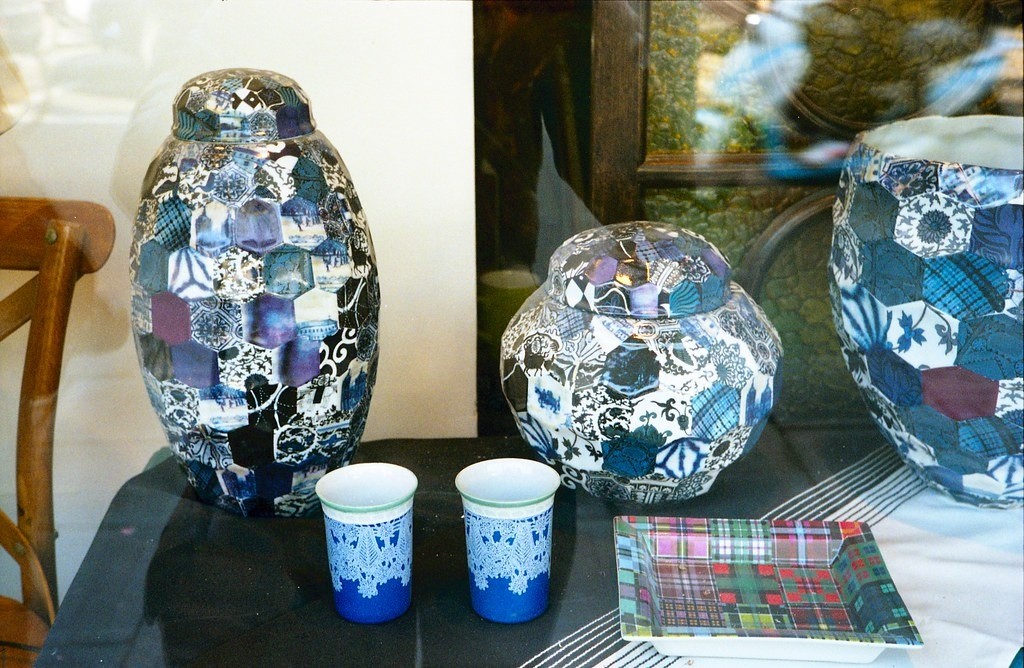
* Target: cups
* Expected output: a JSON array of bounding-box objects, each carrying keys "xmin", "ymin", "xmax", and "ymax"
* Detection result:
[
  {"xmin": 455, "ymin": 457, "xmax": 561, "ymax": 622},
  {"xmin": 828, "ymin": 113, "xmax": 1024, "ymax": 511},
  {"xmin": 315, "ymin": 463, "xmax": 418, "ymax": 625}
]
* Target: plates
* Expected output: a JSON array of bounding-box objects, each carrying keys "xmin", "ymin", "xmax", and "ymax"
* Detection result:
[{"xmin": 612, "ymin": 514, "xmax": 925, "ymax": 665}]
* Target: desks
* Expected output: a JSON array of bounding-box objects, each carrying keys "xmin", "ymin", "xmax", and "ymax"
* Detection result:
[{"xmin": 25, "ymin": 420, "xmax": 891, "ymax": 668}]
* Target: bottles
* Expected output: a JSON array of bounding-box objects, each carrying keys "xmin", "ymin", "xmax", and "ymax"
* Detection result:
[
  {"xmin": 125, "ymin": 71, "xmax": 380, "ymax": 522},
  {"xmin": 499, "ymin": 221, "xmax": 783, "ymax": 512}
]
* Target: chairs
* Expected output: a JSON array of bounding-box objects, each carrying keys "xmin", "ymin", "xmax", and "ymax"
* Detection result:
[{"xmin": 0, "ymin": 196, "xmax": 114, "ymax": 668}]
